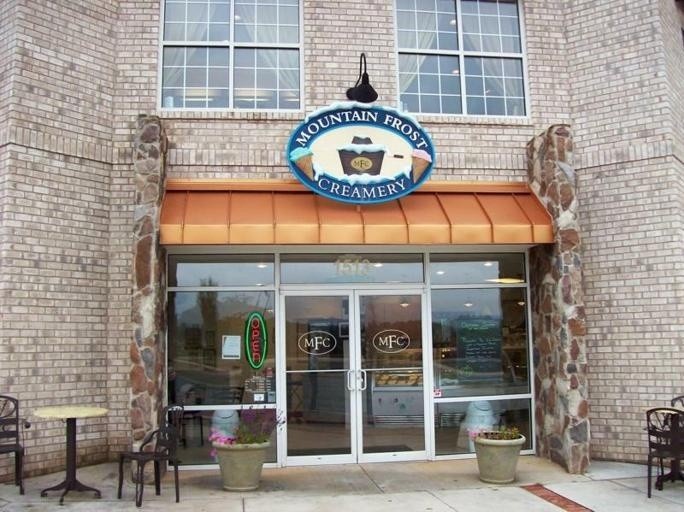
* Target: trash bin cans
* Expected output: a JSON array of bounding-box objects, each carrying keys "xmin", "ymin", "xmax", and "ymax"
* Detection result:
[
  {"xmin": 467, "ymin": 400, "xmax": 493, "ymax": 453},
  {"xmin": 213, "ymin": 410, "xmax": 239, "ymax": 464}
]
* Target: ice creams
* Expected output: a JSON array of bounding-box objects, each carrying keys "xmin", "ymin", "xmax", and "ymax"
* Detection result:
[
  {"xmin": 411, "ymin": 148, "xmax": 432, "ymax": 184},
  {"xmin": 290, "ymin": 147, "xmax": 314, "ymax": 182}
]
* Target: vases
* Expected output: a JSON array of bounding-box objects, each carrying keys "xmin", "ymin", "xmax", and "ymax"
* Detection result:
[
  {"xmin": 211, "ymin": 439, "xmax": 271, "ymax": 491},
  {"xmin": 472, "ymin": 431, "xmax": 526, "ymax": 483}
]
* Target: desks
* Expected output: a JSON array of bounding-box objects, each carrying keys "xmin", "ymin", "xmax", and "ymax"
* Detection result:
[{"xmin": 33, "ymin": 407, "xmax": 109, "ymax": 504}]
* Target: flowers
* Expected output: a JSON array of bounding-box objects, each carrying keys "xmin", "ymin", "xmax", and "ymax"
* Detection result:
[
  {"xmin": 208, "ymin": 406, "xmax": 286, "ymax": 456},
  {"xmin": 469, "ymin": 423, "xmax": 519, "ymax": 442}
]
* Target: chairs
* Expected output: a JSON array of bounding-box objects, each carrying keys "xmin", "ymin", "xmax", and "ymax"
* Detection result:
[
  {"xmin": 644, "ymin": 396, "xmax": 684, "ymax": 499},
  {"xmin": 0, "ymin": 395, "xmax": 31, "ymax": 495},
  {"xmin": 202, "ymin": 387, "xmax": 244, "ymax": 420},
  {"xmin": 176, "ymin": 385, "xmax": 207, "ymax": 449},
  {"xmin": 118, "ymin": 403, "xmax": 184, "ymax": 507}
]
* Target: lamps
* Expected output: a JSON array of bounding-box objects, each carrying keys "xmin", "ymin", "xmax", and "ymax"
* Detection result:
[{"xmin": 346, "ymin": 52, "xmax": 378, "ymax": 104}]
